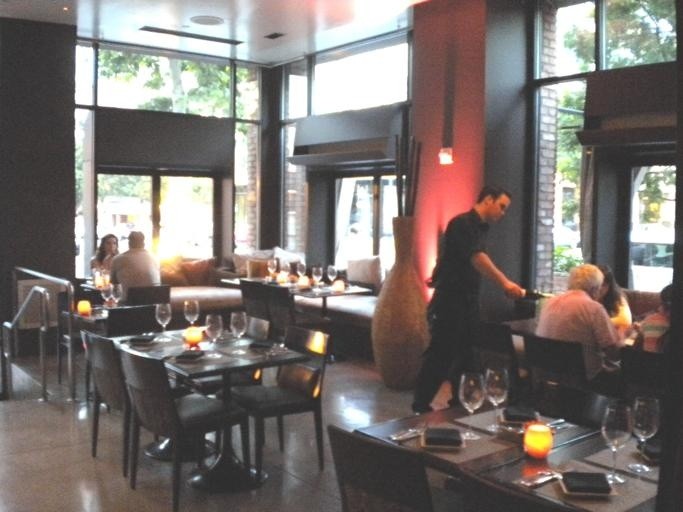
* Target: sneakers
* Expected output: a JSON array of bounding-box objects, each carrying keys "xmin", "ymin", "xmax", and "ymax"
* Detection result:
[
  {"xmin": 448, "ymin": 399, "xmax": 469, "ymax": 409},
  {"xmin": 412, "ymin": 401, "xmax": 433, "ymax": 414}
]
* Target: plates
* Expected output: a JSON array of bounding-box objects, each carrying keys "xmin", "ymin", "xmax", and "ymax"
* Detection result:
[
  {"xmin": 128, "ymin": 336, "xmax": 158, "ymax": 346},
  {"xmin": 175, "ymin": 350, "xmax": 207, "ymax": 364},
  {"xmin": 498, "ymin": 406, "xmax": 542, "ymax": 425},
  {"xmin": 419, "ymin": 432, "xmax": 467, "ymax": 452},
  {"xmin": 557, "ymin": 477, "xmax": 618, "ymax": 499}
]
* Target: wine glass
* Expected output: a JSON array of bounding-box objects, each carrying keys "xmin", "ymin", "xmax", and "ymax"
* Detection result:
[
  {"xmin": 598, "ymin": 397, "xmax": 662, "ymax": 488},
  {"xmin": 266, "ymin": 259, "xmax": 338, "ymax": 294},
  {"xmin": 183, "ymin": 299, "xmax": 199, "ymax": 327},
  {"xmin": 228, "ymin": 311, "xmax": 248, "ymax": 356},
  {"xmin": 99, "ymin": 283, "xmax": 124, "ymax": 303},
  {"xmin": 154, "ymin": 302, "xmax": 173, "ymax": 343},
  {"xmin": 455, "ymin": 367, "xmax": 509, "ymax": 442},
  {"xmin": 205, "ymin": 313, "xmax": 223, "ymax": 359}
]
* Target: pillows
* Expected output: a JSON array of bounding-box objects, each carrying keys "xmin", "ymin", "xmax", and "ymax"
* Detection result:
[{"xmin": 165, "ymin": 248, "xmax": 379, "ymax": 286}]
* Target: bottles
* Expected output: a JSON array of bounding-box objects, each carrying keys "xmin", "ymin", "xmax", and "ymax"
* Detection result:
[{"xmin": 503, "ymin": 289, "xmax": 556, "ymax": 302}]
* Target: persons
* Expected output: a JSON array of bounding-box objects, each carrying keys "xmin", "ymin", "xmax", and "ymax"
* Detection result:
[
  {"xmin": 109, "ymin": 230, "xmax": 162, "ymax": 301},
  {"xmin": 631, "ymin": 284, "xmax": 672, "ymax": 352},
  {"xmin": 412, "ymin": 184, "xmax": 526, "ymax": 412},
  {"xmin": 534, "ymin": 263, "xmax": 624, "ymax": 399},
  {"xmin": 594, "ymin": 263, "xmax": 633, "ymax": 330},
  {"xmin": 89, "ymin": 233, "xmax": 123, "ymax": 274}
]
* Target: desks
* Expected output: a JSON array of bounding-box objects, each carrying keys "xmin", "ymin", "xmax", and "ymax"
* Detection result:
[{"xmin": 102, "ymin": 327, "xmax": 315, "ymax": 494}]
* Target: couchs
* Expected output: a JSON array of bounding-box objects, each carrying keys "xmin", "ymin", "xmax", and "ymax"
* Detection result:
[{"xmin": 73, "ymin": 268, "xmax": 383, "ymax": 350}]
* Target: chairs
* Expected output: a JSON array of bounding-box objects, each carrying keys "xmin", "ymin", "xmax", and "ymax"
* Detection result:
[
  {"xmin": 77, "ymin": 329, "xmax": 164, "ymax": 480},
  {"xmin": 326, "ymin": 371, "xmax": 683, "ymax": 510},
  {"xmin": 215, "ymin": 327, "xmax": 330, "ymax": 480},
  {"xmin": 515, "ymin": 301, "xmax": 683, "ymax": 406},
  {"xmin": 118, "ymin": 347, "xmax": 250, "ymax": 511}
]
